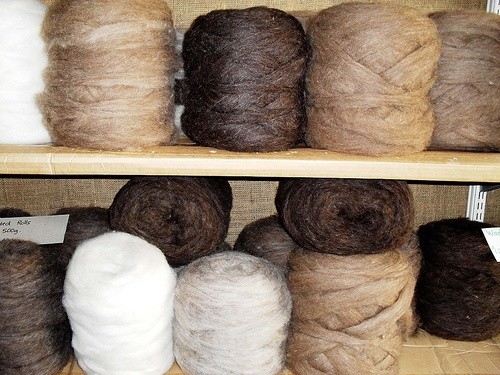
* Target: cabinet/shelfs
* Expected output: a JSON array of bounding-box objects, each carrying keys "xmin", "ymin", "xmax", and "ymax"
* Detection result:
[{"xmin": 0, "ymin": 0, "xmax": 500, "ymax": 375}]
